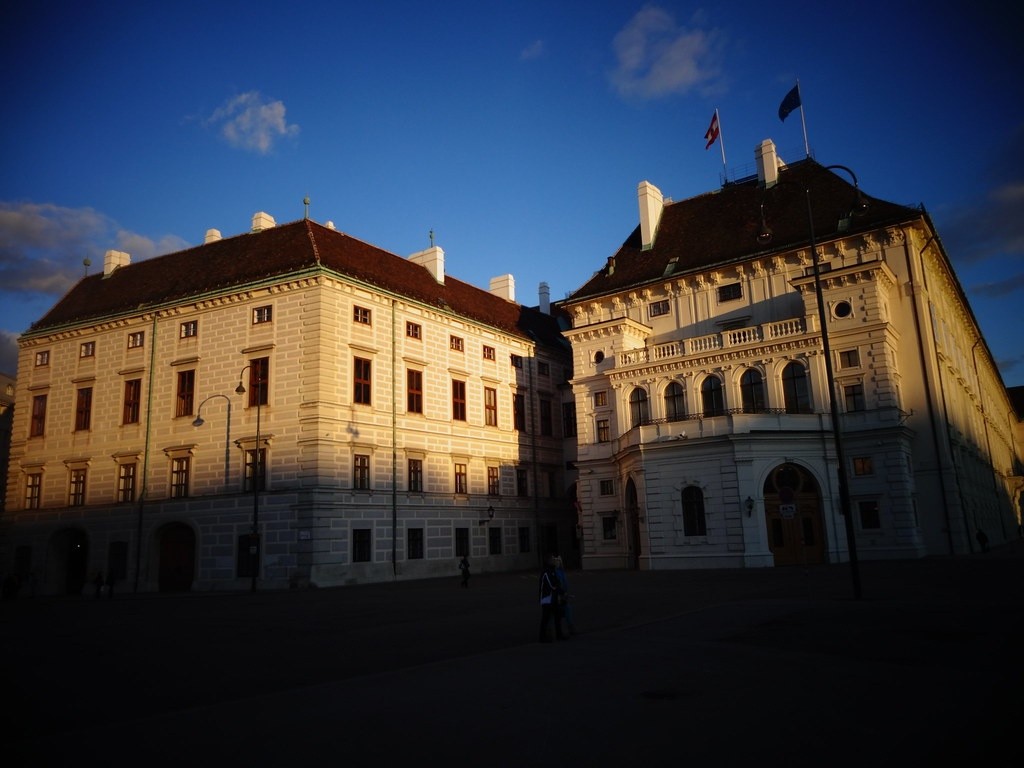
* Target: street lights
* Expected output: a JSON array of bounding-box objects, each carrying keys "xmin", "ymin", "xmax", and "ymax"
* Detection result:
[
  {"xmin": 235, "ymin": 365, "xmax": 262, "ymax": 591},
  {"xmin": 754, "ymin": 164, "xmax": 872, "ymax": 600}
]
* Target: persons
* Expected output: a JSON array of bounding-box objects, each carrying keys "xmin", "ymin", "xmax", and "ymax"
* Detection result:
[
  {"xmin": 975, "ymin": 528, "xmax": 990, "ymax": 550},
  {"xmin": 539, "ymin": 556, "xmax": 570, "ymax": 644},
  {"xmin": 551, "ymin": 555, "xmax": 582, "ymax": 635},
  {"xmin": 459, "ymin": 555, "xmax": 471, "ymax": 588},
  {"xmin": 93, "ymin": 570, "xmax": 117, "ymax": 601},
  {"xmin": 1018, "ymin": 525, "xmax": 1023, "ymax": 538}
]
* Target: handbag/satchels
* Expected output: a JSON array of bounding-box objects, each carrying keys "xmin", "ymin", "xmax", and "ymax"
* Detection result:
[{"xmin": 459, "ymin": 564, "xmax": 465, "ymax": 569}]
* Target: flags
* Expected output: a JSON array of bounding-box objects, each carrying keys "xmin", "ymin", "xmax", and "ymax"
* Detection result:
[
  {"xmin": 778, "ymin": 84, "xmax": 802, "ymax": 123},
  {"xmin": 704, "ymin": 111, "xmax": 719, "ymax": 150}
]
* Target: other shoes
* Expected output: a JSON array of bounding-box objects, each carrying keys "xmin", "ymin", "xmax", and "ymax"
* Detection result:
[
  {"xmin": 569, "ymin": 623, "xmax": 582, "ymax": 634},
  {"xmin": 556, "ymin": 634, "xmax": 570, "ymax": 642}
]
